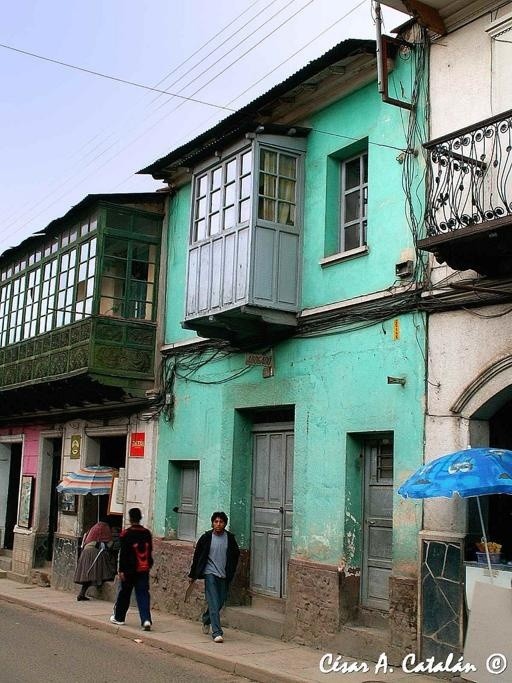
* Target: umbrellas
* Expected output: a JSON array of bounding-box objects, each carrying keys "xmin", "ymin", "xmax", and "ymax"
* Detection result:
[
  {"xmin": 56, "ymin": 464, "xmax": 120, "ymax": 522},
  {"xmin": 397, "ymin": 443, "xmax": 511, "ymax": 584}
]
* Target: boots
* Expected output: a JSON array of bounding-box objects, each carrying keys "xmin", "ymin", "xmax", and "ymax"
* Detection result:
[{"xmin": 77, "ymin": 584, "xmax": 90, "ymax": 601}]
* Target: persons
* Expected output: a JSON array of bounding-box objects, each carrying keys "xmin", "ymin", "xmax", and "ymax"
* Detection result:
[
  {"xmin": 109, "ymin": 507, "xmax": 157, "ymax": 631},
  {"xmin": 74, "ymin": 517, "xmax": 116, "ymax": 600},
  {"xmin": 187, "ymin": 510, "xmax": 241, "ymax": 643}
]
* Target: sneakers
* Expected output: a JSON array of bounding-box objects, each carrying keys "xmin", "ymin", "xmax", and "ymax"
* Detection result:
[
  {"xmin": 142, "ymin": 620, "xmax": 151, "ymax": 631},
  {"xmin": 201, "ymin": 613, "xmax": 210, "ymax": 633},
  {"xmin": 214, "ymin": 636, "xmax": 224, "ymax": 642},
  {"xmin": 110, "ymin": 615, "xmax": 125, "ymax": 625}
]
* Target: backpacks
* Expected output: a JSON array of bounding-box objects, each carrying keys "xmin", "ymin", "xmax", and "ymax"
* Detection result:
[{"xmin": 127, "ymin": 528, "xmax": 149, "ymax": 572}]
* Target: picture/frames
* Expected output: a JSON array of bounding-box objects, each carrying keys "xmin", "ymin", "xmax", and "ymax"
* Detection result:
[
  {"xmin": 18, "ymin": 474, "xmax": 35, "ymax": 528},
  {"xmin": 57, "ymin": 478, "xmax": 78, "ymax": 512},
  {"xmin": 107, "ymin": 472, "xmax": 124, "ymax": 515}
]
